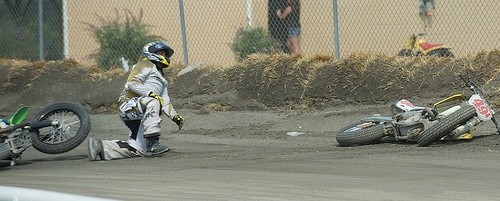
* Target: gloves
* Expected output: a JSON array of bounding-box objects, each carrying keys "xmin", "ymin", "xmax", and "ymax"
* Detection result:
[
  {"xmin": 173, "ymin": 116, "xmax": 184, "ymax": 130},
  {"xmin": 150, "ymin": 93, "xmax": 164, "ymax": 107}
]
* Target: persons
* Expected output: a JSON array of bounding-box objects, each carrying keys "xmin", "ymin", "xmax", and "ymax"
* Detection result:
[
  {"xmin": 88, "ymin": 41, "xmax": 185, "ymax": 161},
  {"xmin": 276, "ymin": 0, "xmax": 301, "ymax": 57},
  {"xmin": 418, "ymin": 0, "xmax": 434, "ymax": 43}
]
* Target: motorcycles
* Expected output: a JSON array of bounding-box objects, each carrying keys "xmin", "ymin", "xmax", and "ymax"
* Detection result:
[
  {"xmin": 0, "ymin": 102, "xmax": 91, "ymax": 168},
  {"xmin": 336, "ymin": 76, "xmax": 500, "ymax": 147},
  {"xmin": 398, "ymin": 34, "xmax": 454, "ymax": 58}
]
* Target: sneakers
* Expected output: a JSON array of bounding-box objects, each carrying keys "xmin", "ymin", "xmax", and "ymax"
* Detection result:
[
  {"xmin": 146, "ymin": 139, "xmax": 168, "ymax": 156},
  {"xmin": 88, "ymin": 137, "xmax": 102, "ymax": 160}
]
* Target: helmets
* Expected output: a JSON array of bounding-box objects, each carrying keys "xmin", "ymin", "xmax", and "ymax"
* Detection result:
[{"xmin": 142, "ymin": 41, "xmax": 174, "ymax": 67}]
